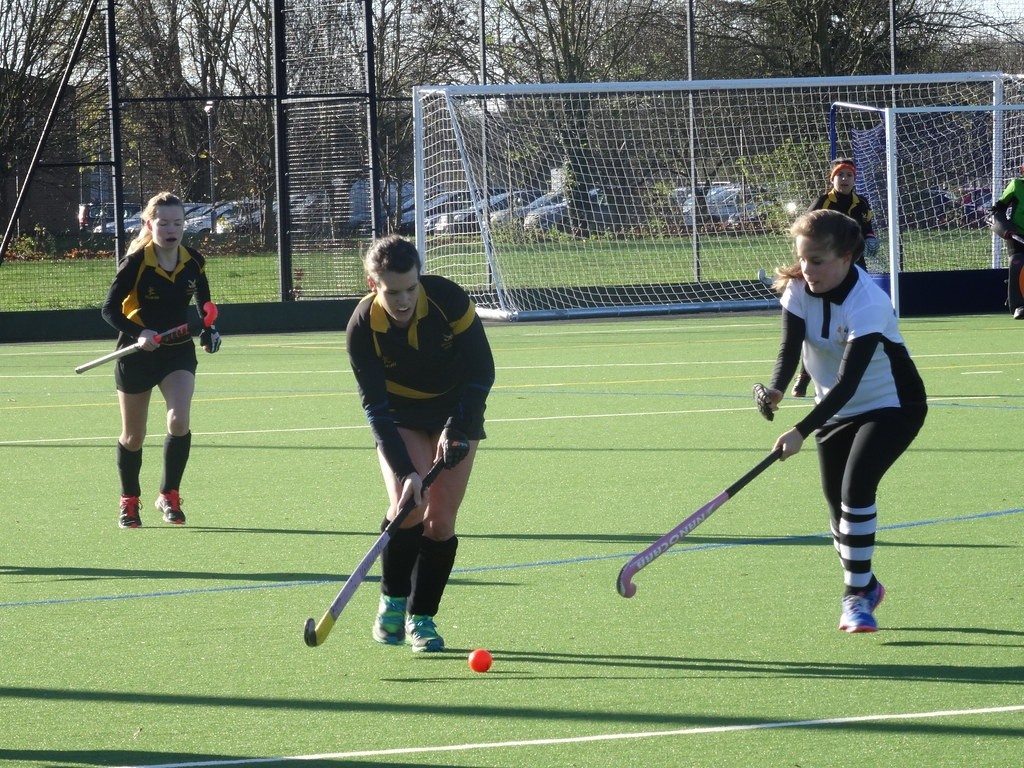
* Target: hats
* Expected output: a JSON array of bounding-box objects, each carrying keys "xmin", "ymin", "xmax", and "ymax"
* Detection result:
[{"xmin": 829, "ymin": 158, "xmax": 858, "ymax": 182}]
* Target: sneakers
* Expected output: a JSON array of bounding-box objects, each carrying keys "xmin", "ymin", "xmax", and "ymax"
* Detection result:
[
  {"xmin": 117, "ymin": 495, "xmax": 143, "ymax": 529},
  {"xmin": 1014, "ymin": 306, "xmax": 1024, "ymax": 319},
  {"xmin": 372, "ymin": 593, "xmax": 407, "ymax": 645},
  {"xmin": 839, "ymin": 581, "xmax": 885, "ymax": 632},
  {"xmin": 791, "ymin": 371, "xmax": 812, "ymax": 397},
  {"xmin": 154, "ymin": 488, "xmax": 186, "ymax": 524},
  {"xmin": 405, "ymin": 613, "xmax": 445, "ymax": 653}
]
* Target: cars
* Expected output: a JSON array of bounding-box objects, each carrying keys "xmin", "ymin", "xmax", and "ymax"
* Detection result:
[
  {"xmin": 77, "ymin": 194, "xmax": 310, "ymax": 238},
  {"xmin": 393, "ymin": 182, "xmax": 767, "ymax": 237},
  {"xmin": 899, "ymin": 186, "xmax": 993, "ymax": 231}
]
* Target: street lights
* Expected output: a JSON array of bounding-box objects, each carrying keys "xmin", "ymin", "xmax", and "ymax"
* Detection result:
[{"xmin": 204, "ymin": 99, "xmax": 217, "ymax": 235}]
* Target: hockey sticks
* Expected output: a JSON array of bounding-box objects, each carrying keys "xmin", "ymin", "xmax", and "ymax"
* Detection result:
[
  {"xmin": 615, "ymin": 446, "xmax": 784, "ymax": 598},
  {"xmin": 304, "ymin": 458, "xmax": 443, "ymax": 648},
  {"xmin": 985, "ymin": 220, "xmax": 1024, "ymax": 244},
  {"xmin": 74, "ymin": 301, "xmax": 219, "ymax": 375}
]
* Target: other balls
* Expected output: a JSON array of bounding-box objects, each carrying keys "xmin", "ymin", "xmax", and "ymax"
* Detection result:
[{"xmin": 468, "ymin": 649, "xmax": 492, "ymax": 672}]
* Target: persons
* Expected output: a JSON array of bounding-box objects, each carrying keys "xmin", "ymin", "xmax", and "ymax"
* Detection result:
[
  {"xmin": 752, "ymin": 209, "xmax": 929, "ymax": 633},
  {"xmin": 345, "ymin": 235, "xmax": 495, "ymax": 653},
  {"xmin": 992, "ymin": 159, "xmax": 1024, "ymax": 320},
  {"xmin": 101, "ymin": 192, "xmax": 222, "ymax": 528},
  {"xmin": 792, "ymin": 157, "xmax": 879, "ymax": 398}
]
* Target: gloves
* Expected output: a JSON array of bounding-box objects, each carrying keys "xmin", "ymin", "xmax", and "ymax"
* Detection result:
[
  {"xmin": 199, "ymin": 324, "xmax": 222, "ymax": 354},
  {"xmin": 862, "ymin": 234, "xmax": 880, "ymax": 257}
]
